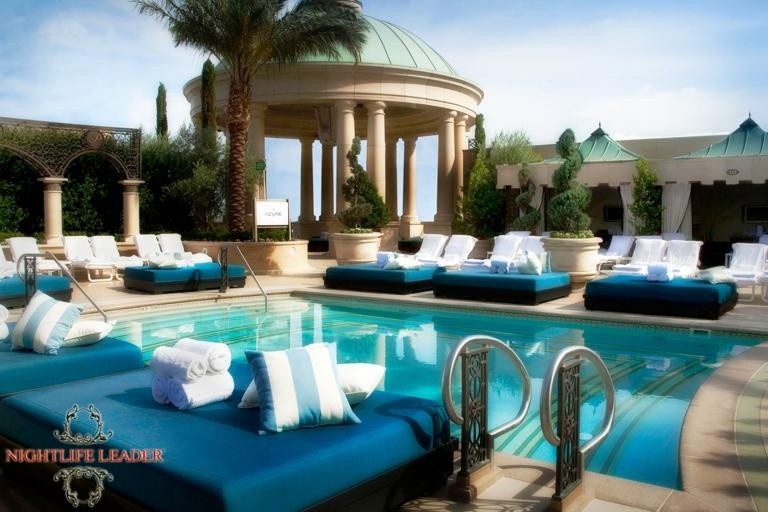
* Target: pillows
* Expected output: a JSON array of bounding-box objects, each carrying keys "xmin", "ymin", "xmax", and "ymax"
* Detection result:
[
  {"xmin": 385, "ymin": 259, "xmax": 424, "ymax": 270},
  {"xmin": 60, "ymin": 316, "xmax": 119, "ymax": 347},
  {"xmin": 516, "ymin": 246, "xmax": 541, "ymax": 276},
  {"xmin": 701, "ymin": 263, "xmax": 737, "ymax": 286},
  {"xmin": 245, "ymin": 341, "xmax": 363, "ymax": 439},
  {"xmin": 148, "ymin": 256, "xmax": 181, "ymax": 267},
  {"xmin": 236, "ymin": 356, "xmax": 387, "ymax": 412},
  {"xmin": 9, "ymin": 288, "xmax": 85, "ymax": 356}
]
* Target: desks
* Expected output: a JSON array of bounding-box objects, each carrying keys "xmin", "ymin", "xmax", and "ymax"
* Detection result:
[{"xmin": 45, "ymin": 259, "xmax": 71, "ymax": 278}]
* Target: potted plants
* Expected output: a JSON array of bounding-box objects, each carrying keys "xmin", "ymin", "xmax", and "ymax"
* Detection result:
[
  {"xmin": 332, "ymin": 134, "xmax": 393, "ymax": 267},
  {"xmin": 543, "ymin": 125, "xmax": 602, "ymax": 287},
  {"xmin": 454, "ymin": 138, "xmax": 497, "ymax": 262}
]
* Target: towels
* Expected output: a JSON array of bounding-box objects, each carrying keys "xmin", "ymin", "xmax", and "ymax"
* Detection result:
[
  {"xmin": 646, "ymin": 262, "xmax": 673, "ymax": 282},
  {"xmin": 489, "ymin": 254, "xmax": 512, "ymax": 275},
  {"xmin": 0, "ymin": 302, "xmax": 13, "ymax": 340},
  {"xmin": 375, "ymin": 251, "xmax": 392, "ymax": 267},
  {"xmin": 646, "ymin": 358, "xmax": 671, "ymax": 370},
  {"xmin": 150, "ymin": 336, "xmax": 235, "ymax": 412}
]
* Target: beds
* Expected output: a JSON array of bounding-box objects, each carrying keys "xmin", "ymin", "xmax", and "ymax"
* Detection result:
[
  {"xmin": 122, "ymin": 264, "xmax": 247, "ymax": 295},
  {"xmin": 431, "ymin": 270, "xmax": 572, "ymax": 306},
  {"xmin": 322, "ymin": 257, "xmax": 447, "ymax": 296},
  {"xmin": 0, "ymin": 320, "xmax": 146, "ymax": 405},
  {"xmin": 582, "ymin": 273, "xmax": 740, "ymax": 321},
  {"xmin": 0, "ymin": 274, "xmax": 73, "ymax": 311},
  {"xmin": 0, "ymin": 363, "xmax": 460, "ymax": 512}
]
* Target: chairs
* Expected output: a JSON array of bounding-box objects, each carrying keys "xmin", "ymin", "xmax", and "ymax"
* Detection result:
[
  {"xmin": 132, "ymin": 233, "xmax": 180, "ymax": 267},
  {"xmin": 398, "ymin": 234, "xmax": 450, "ymax": 265},
  {"xmin": 63, "ymin": 233, "xmax": 117, "ymax": 282},
  {"xmin": 417, "ymin": 234, "xmax": 479, "ymax": 272},
  {"xmin": 596, "ymin": 233, "xmax": 705, "ymax": 278},
  {"xmin": 461, "ymin": 229, "xmax": 554, "ymax": 272},
  {"xmin": 0, "ymin": 248, "xmax": 23, "ymax": 274},
  {"xmin": 89, "ymin": 234, "xmax": 146, "ymax": 281},
  {"xmin": 4, "ymin": 235, "xmax": 72, "ymax": 278},
  {"xmin": 716, "ymin": 234, "xmax": 768, "ymax": 305},
  {"xmin": 155, "ymin": 233, "xmax": 214, "ymax": 265}
]
